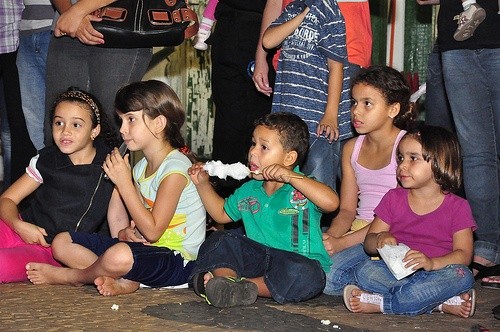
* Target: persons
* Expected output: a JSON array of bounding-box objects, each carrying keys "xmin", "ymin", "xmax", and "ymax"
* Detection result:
[
  {"xmin": 319, "ymin": 64, "xmax": 425, "ymax": 296},
  {"xmin": 0, "ymin": 86, "xmax": 135, "ymax": 283},
  {"xmin": 252, "ymin": 0, "xmax": 372, "ymax": 96},
  {"xmin": 187, "ymin": 112, "xmax": 340, "ymax": 308},
  {"xmin": 0, "ymin": 0, "xmax": 58, "ymax": 194},
  {"xmin": 25, "ymin": 80, "xmax": 206, "ymax": 297},
  {"xmin": 416, "ymin": 0, "xmax": 500, "ymax": 289},
  {"xmin": 42, "ymin": 0, "xmax": 155, "ymax": 169},
  {"xmin": 416, "ymin": 0, "xmax": 486, "ymax": 43},
  {"xmin": 262, "ymin": 0, "xmax": 353, "ymax": 228},
  {"xmin": 191, "ymin": 0, "xmax": 218, "ymax": 51},
  {"xmin": 341, "ymin": 125, "xmax": 480, "ymax": 318},
  {"xmin": 207, "ymin": 0, "xmax": 279, "ymax": 239}
]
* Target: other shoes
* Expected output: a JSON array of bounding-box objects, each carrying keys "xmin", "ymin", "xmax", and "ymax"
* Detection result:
[
  {"xmin": 454, "ymin": 3, "xmax": 486, "ymax": 43},
  {"xmin": 192, "ymin": 17, "xmax": 214, "ymax": 51}
]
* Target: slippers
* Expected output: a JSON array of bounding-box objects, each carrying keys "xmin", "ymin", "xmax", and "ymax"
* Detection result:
[
  {"xmin": 468, "ymin": 261, "xmax": 500, "ymax": 281},
  {"xmin": 480, "ymin": 273, "xmax": 500, "ymax": 289},
  {"xmin": 438, "ymin": 288, "xmax": 476, "ymax": 319},
  {"xmin": 343, "ymin": 284, "xmax": 384, "ymax": 314}
]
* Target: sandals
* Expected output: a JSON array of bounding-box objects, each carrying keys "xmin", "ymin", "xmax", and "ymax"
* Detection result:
[
  {"xmin": 205, "ymin": 274, "xmax": 259, "ymax": 308},
  {"xmin": 193, "ymin": 271, "xmax": 214, "ymax": 299}
]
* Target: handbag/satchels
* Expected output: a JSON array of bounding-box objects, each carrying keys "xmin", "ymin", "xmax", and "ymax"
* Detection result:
[{"xmin": 85, "ymin": 0, "xmax": 200, "ymax": 48}]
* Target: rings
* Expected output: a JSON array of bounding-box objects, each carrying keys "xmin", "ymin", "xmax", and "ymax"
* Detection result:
[
  {"xmin": 255, "ymin": 85, "xmax": 258, "ymax": 88},
  {"xmin": 60, "ymin": 32, "xmax": 67, "ymax": 35}
]
